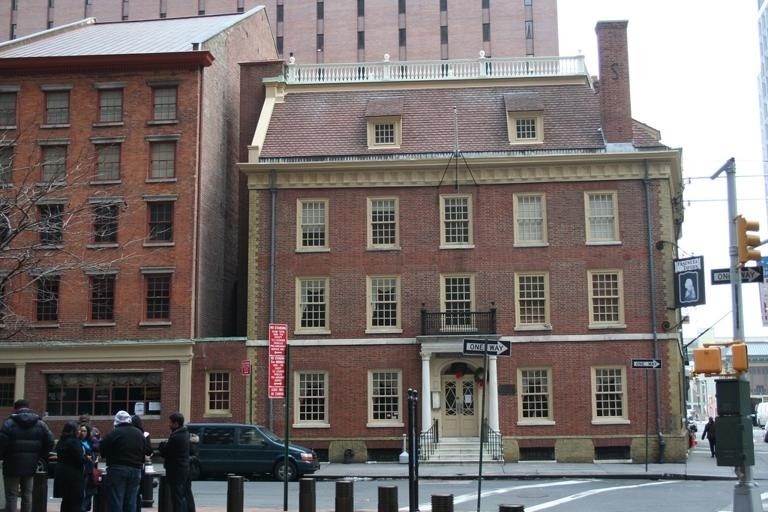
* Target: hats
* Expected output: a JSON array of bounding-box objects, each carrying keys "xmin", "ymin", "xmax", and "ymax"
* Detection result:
[{"xmin": 113, "ymin": 410, "xmax": 132, "ymax": 426}]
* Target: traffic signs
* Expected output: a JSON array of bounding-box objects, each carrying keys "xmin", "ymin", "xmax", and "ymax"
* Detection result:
[
  {"xmin": 631, "ymin": 358, "xmax": 662, "ymax": 369},
  {"xmin": 711, "ymin": 266, "xmax": 763, "ymax": 285},
  {"xmin": 462, "ymin": 338, "xmax": 511, "ymax": 357}
]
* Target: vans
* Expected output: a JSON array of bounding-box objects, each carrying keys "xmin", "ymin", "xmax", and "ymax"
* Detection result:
[{"xmin": 185, "ymin": 423, "xmax": 320, "ymax": 481}]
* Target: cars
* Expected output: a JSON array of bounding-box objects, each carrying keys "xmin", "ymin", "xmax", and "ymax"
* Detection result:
[{"xmin": 751, "ymin": 395, "xmax": 767, "ymax": 441}]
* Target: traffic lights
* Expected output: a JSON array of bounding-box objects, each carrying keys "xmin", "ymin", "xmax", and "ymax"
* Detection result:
[{"xmin": 736, "ymin": 217, "xmax": 761, "ymax": 263}]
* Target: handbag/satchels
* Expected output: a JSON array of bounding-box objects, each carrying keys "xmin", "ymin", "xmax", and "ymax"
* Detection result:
[{"xmin": 48, "ymin": 454, "xmax": 76, "ymax": 473}]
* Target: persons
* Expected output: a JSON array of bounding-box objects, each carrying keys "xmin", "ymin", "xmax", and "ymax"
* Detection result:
[
  {"xmin": 0, "ymin": 399, "xmax": 201, "ymax": 512},
  {"xmin": 701, "ymin": 416, "xmax": 718, "ymax": 458}
]
